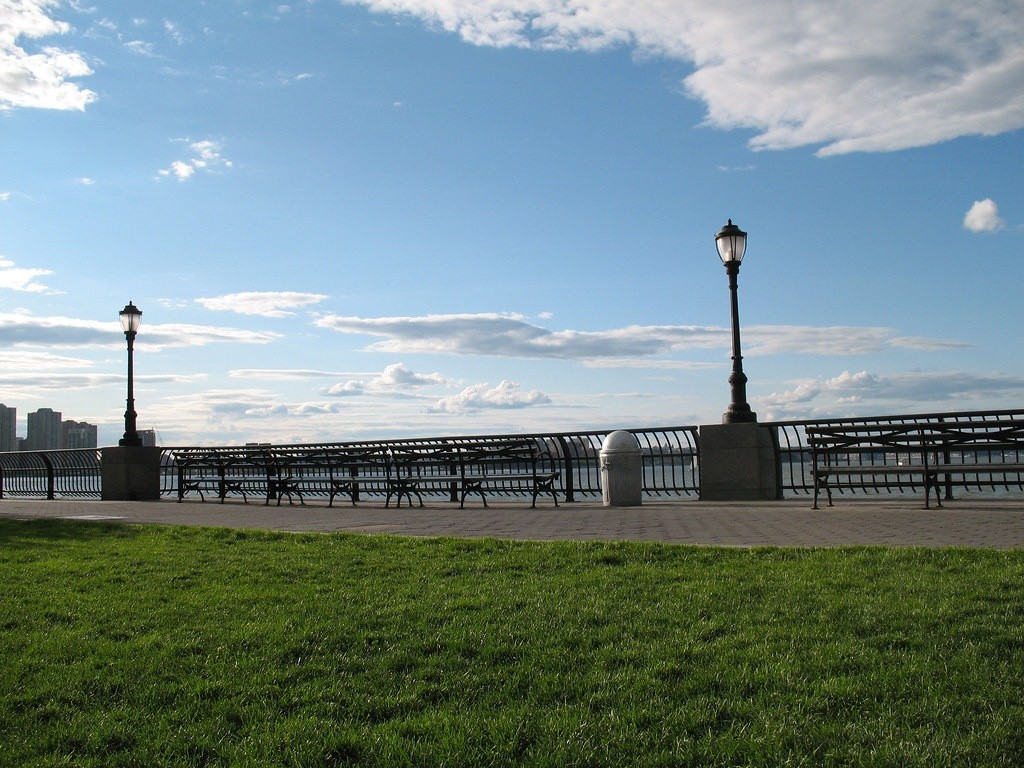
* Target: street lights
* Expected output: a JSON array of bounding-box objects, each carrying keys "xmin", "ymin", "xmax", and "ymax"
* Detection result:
[
  {"xmin": 118, "ymin": 300, "xmax": 143, "ymax": 446},
  {"xmin": 713, "ymin": 217, "xmax": 758, "ymax": 424}
]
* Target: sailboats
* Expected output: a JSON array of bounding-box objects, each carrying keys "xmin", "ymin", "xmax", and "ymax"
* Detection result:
[{"xmin": 688, "ymin": 450, "xmax": 698, "ymax": 471}]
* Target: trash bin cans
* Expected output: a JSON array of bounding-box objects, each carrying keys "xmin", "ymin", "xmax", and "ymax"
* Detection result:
[{"xmin": 598, "ymin": 431, "xmax": 644, "ymax": 507}]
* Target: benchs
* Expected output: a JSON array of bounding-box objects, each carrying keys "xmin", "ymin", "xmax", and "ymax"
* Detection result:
[
  {"xmin": 172, "ymin": 439, "xmax": 560, "ymax": 510},
  {"xmin": 805, "ymin": 419, "xmax": 1024, "ymax": 509}
]
